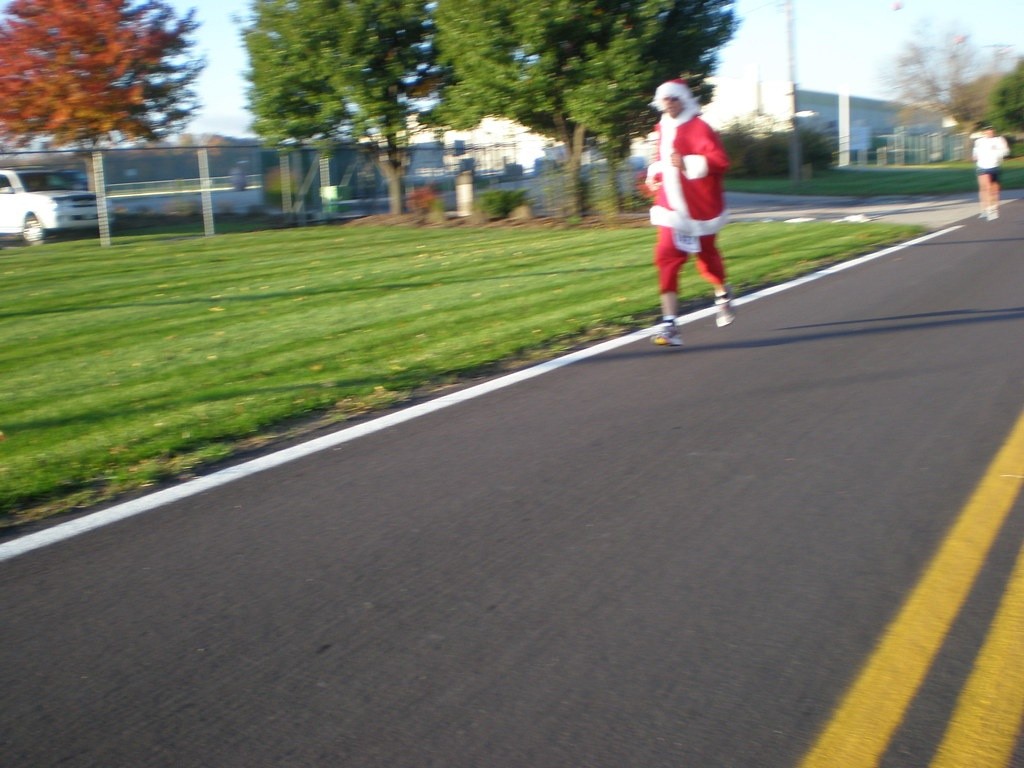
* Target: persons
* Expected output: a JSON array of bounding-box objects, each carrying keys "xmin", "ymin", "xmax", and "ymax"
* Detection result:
[
  {"xmin": 645, "ymin": 79, "xmax": 735, "ymax": 346},
  {"xmin": 228, "ymin": 159, "xmax": 247, "ymax": 190},
  {"xmin": 972, "ymin": 125, "xmax": 1011, "ymax": 222}
]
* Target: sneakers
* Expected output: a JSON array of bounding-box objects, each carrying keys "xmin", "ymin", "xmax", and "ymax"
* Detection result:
[
  {"xmin": 715, "ymin": 296, "xmax": 736, "ymax": 327},
  {"xmin": 651, "ymin": 320, "xmax": 684, "ymax": 347}
]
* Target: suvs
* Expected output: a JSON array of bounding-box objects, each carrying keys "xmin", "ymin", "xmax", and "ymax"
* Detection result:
[{"xmin": 0, "ymin": 167, "xmax": 118, "ymax": 247}]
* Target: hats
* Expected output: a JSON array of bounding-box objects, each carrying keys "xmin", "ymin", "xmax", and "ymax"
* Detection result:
[{"xmin": 655, "ymin": 77, "xmax": 693, "ymax": 111}]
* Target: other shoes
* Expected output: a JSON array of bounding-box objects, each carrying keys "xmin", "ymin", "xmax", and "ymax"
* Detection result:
[{"xmin": 979, "ymin": 205, "xmax": 1000, "ymax": 222}]
